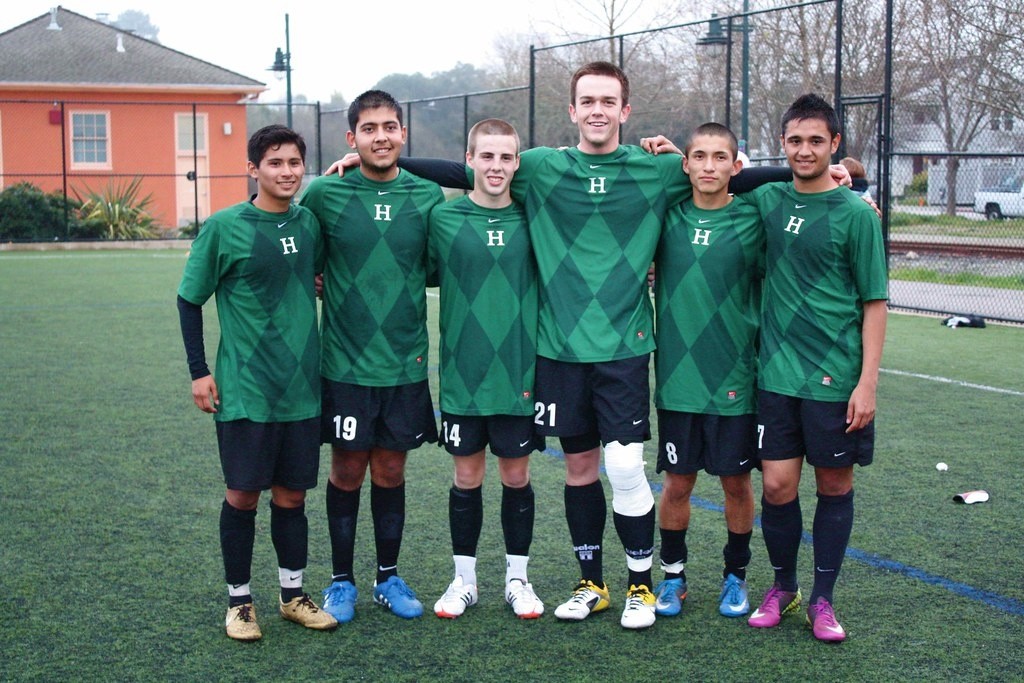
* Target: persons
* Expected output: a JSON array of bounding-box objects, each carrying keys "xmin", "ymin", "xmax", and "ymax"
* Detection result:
[
  {"xmin": 296, "ymin": 89, "xmax": 446, "ymax": 621},
  {"xmin": 638, "ymin": 94, "xmax": 889, "ymax": 642},
  {"xmin": 316, "ymin": 118, "xmax": 654, "ymax": 618},
  {"xmin": 838, "ymin": 156, "xmax": 872, "ymax": 198},
  {"xmin": 736, "ymin": 149, "xmax": 751, "ymax": 168},
  {"xmin": 178, "ymin": 124, "xmax": 339, "ymax": 639},
  {"xmin": 320, "ymin": 60, "xmax": 852, "ymax": 628},
  {"xmin": 651, "ymin": 123, "xmax": 883, "ymax": 617}
]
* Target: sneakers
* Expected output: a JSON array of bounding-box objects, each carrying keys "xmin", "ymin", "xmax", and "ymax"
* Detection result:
[
  {"xmin": 717, "ymin": 573, "xmax": 750, "ymax": 618},
  {"xmin": 225, "ymin": 601, "xmax": 263, "ymax": 640},
  {"xmin": 434, "ymin": 575, "xmax": 478, "ymax": 619},
  {"xmin": 373, "ymin": 575, "xmax": 424, "ymax": 617},
  {"xmin": 654, "ymin": 578, "xmax": 688, "ymax": 616},
  {"xmin": 505, "ymin": 578, "xmax": 544, "ymax": 618},
  {"xmin": 748, "ymin": 584, "xmax": 802, "ymax": 628},
  {"xmin": 279, "ymin": 591, "xmax": 339, "ymax": 630},
  {"xmin": 806, "ymin": 595, "xmax": 846, "ymax": 641},
  {"xmin": 620, "ymin": 583, "xmax": 657, "ymax": 628},
  {"xmin": 554, "ymin": 579, "xmax": 611, "ymax": 620},
  {"xmin": 322, "ymin": 580, "xmax": 358, "ymax": 623}
]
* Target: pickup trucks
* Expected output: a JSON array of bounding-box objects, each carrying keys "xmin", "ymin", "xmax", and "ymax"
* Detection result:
[{"xmin": 974, "ymin": 184, "xmax": 1024, "ymax": 222}]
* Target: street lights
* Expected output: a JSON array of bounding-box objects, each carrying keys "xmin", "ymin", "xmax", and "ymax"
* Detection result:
[
  {"xmin": 266, "ymin": 14, "xmax": 293, "ymax": 128},
  {"xmin": 695, "ymin": 0, "xmax": 753, "ymax": 156}
]
